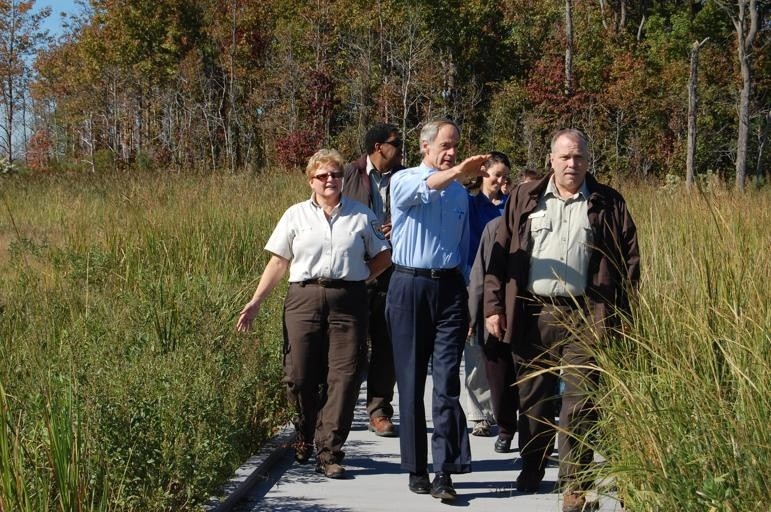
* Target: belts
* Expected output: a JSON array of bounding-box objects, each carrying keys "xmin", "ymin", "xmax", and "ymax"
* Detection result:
[
  {"xmin": 395, "ymin": 265, "xmax": 461, "ymax": 278},
  {"xmin": 289, "ymin": 279, "xmax": 366, "ymax": 289},
  {"xmin": 527, "ymin": 295, "xmax": 585, "ymax": 307}
]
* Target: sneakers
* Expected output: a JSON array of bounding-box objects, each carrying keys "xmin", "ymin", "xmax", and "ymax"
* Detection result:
[
  {"xmin": 471, "ymin": 416, "xmax": 597, "ymax": 510},
  {"xmin": 296, "ymin": 442, "xmax": 314, "ymax": 462},
  {"xmin": 369, "ymin": 416, "xmax": 394, "ymax": 436},
  {"xmin": 315, "ymin": 462, "xmax": 345, "ymax": 478}
]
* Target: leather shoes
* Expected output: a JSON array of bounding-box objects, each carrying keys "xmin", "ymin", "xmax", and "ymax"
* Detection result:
[
  {"xmin": 431, "ymin": 474, "xmax": 456, "ymax": 499},
  {"xmin": 409, "ymin": 472, "xmax": 432, "ymax": 494}
]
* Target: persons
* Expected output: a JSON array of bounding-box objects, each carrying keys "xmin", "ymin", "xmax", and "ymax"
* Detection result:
[
  {"xmin": 339, "ymin": 123, "xmax": 403, "ymax": 436},
  {"xmin": 383, "ymin": 119, "xmax": 492, "ymax": 498},
  {"xmin": 482, "ymin": 130, "xmax": 642, "ymax": 511},
  {"xmin": 467, "ymin": 169, "xmax": 544, "ymax": 453},
  {"xmin": 464, "ymin": 151, "xmax": 513, "ymax": 436},
  {"xmin": 236, "ymin": 147, "xmax": 391, "ymax": 478}
]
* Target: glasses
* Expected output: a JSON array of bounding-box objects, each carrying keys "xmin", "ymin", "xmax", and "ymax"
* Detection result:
[
  {"xmin": 311, "ymin": 172, "xmax": 343, "ymax": 178},
  {"xmin": 384, "ymin": 139, "xmax": 403, "ymax": 148}
]
